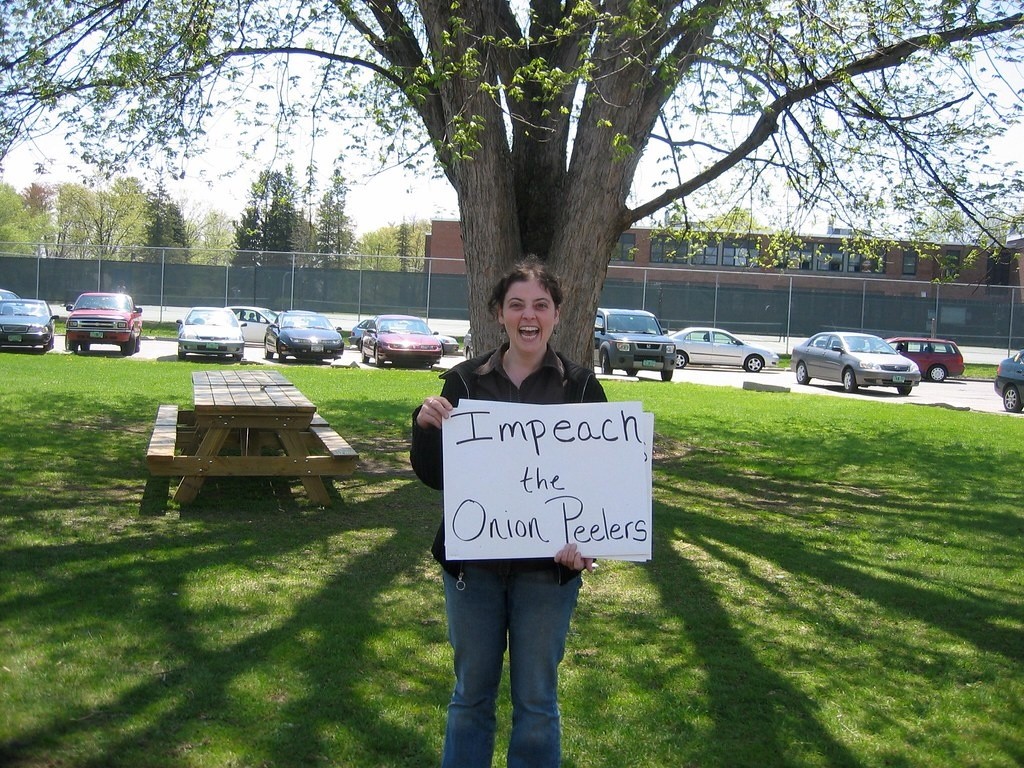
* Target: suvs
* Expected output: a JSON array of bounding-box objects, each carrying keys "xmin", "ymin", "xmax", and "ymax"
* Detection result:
[{"xmin": 593, "ymin": 308, "xmax": 676, "ymax": 381}]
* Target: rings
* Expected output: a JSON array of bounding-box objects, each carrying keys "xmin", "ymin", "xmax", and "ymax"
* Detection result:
[{"xmin": 430, "ymin": 399, "xmax": 433, "ymax": 404}]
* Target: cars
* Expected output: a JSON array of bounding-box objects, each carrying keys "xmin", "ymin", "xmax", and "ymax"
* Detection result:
[
  {"xmin": 176, "ymin": 307, "xmax": 247, "ymax": 361},
  {"xmin": 994, "ymin": 349, "xmax": 1024, "ymax": 413},
  {"xmin": 349, "ymin": 319, "xmax": 459, "ymax": 356},
  {"xmin": 0, "ymin": 290, "xmax": 24, "ymax": 308},
  {"xmin": 360, "ymin": 314, "xmax": 443, "ymax": 369},
  {"xmin": 877, "ymin": 336, "xmax": 965, "ymax": 383},
  {"xmin": 0, "ymin": 299, "xmax": 59, "ymax": 351},
  {"xmin": 463, "ymin": 329, "xmax": 473, "ymax": 360},
  {"xmin": 789, "ymin": 332, "xmax": 921, "ymax": 396},
  {"xmin": 264, "ymin": 310, "xmax": 344, "ymax": 363},
  {"xmin": 669, "ymin": 327, "xmax": 779, "ymax": 373},
  {"xmin": 224, "ymin": 306, "xmax": 278, "ymax": 343}
]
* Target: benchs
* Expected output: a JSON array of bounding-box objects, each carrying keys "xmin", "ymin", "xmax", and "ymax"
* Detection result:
[
  {"xmin": 146, "ymin": 404, "xmax": 178, "ymax": 476},
  {"xmin": 309, "ymin": 411, "xmax": 358, "ymax": 477}
]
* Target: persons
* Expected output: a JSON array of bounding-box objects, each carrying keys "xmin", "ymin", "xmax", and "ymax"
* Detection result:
[
  {"xmin": 249, "ymin": 313, "xmax": 256, "ymax": 321},
  {"xmin": 410, "ymin": 265, "xmax": 610, "ymax": 768}
]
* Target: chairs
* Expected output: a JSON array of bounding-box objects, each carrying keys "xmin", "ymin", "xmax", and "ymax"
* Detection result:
[
  {"xmin": 248, "ymin": 313, "xmax": 256, "ymax": 322},
  {"xmin": 832, "ymin": 341, "xmax": 841, "ymax": 347},
  {"xmin": 816, "ymin": 340, "xmax": 826, "ymax": 346}
]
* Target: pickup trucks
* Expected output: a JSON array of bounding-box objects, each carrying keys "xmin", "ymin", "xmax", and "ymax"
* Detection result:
[{"xmin": 65, "ymin": 292, "xmax": 143, "ymax": 356}]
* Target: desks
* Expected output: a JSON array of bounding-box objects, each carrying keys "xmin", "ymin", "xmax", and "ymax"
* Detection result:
[{"xmin": 172, "ymin": 368, "xmax": 333, "ymax": 506}]
